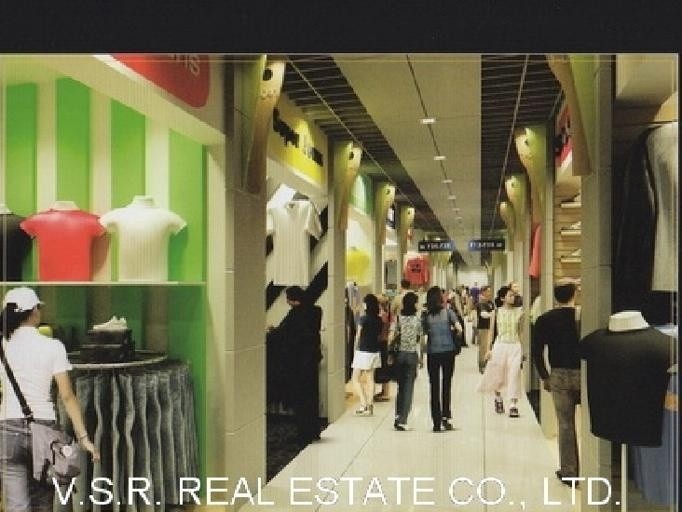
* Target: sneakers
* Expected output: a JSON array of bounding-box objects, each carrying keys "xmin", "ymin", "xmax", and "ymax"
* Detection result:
[
  {"xmin": 93, "ymin": 314, "xmax": 127, "ymax": 330},
  {"xmin": 556, "ymin": 470, "xmax": 577, "ymax": 487},
  {"xmin": 355, "ymin": 392, "xmax": 453, "ymax": 432},
  {"xmin": 494, "ymin": 397, "xmax": 503, "ymax": 412},
  {"xmin": 510, "ymin": 404, "xmax": 519, "ymax": 417}
]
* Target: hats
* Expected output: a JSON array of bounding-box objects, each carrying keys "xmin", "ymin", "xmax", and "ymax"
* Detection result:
[{"xmin": 4, "ymin": 288, "xmax": 47, "ymax": 312}]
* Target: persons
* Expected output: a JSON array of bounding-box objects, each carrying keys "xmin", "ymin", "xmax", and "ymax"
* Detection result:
[
  {"xmin": 0, "ymin": 286, "xmax": 101, "ymax": 512},
  {"xmin": 268, "ymin": 286, "xmax": 320, "ymax": 441},
  {"xmin": 20, "ymin": 201, "xmax": 106, "ymax": 283},
  {"xmin": 532, "ymin": 281, "xmax": 580, "ymax": 487},
  {"xmin": 99, "ymin": 194, "xmax": 187, "ymax": 282},
  {"xmin": 576, "ymin": 308, "xmax": 679, "ymax": 446},
  {"xmin": 0, "ymin": 204, "xmax": 30, "ymax": 280},
  {"xmin": 351, "ymin": 279, "xmax": 528, "ymax": 430}
]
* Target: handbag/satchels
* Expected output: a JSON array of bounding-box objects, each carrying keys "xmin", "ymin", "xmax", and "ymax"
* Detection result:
[
  {"xmin": 28, "ymin": 421, "xmax": 81, "ymax": 486},
  {"xmin": 447, "ymin": 309, "xmax": 461, "ymax": 354},
  {"xmin": 492, "ymin": 310, "xmax": 498, "ymax": 344},
  {"xmin": 389, "ymin": 315, "xmax": 401, "ymax": 352}
]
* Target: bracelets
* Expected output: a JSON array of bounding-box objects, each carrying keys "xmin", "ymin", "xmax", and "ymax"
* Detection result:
[{"xmin": 78, "ymin": 433, "xmax": 89, "ymax": 440}]
